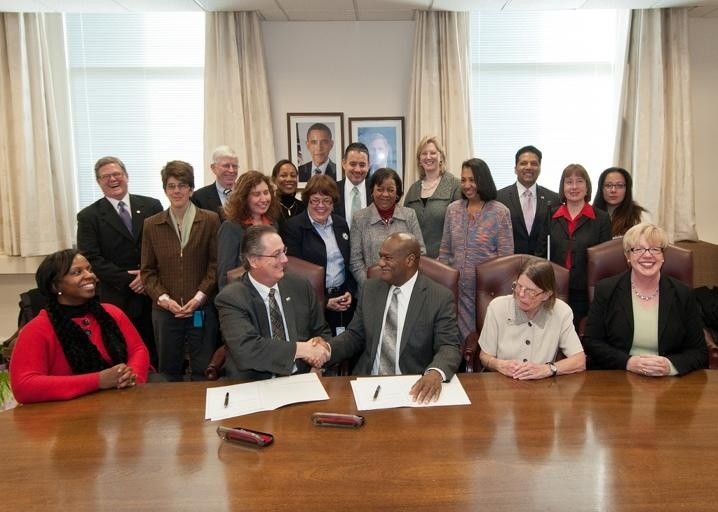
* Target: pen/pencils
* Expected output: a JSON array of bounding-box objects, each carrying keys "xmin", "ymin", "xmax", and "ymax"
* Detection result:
[
  {"xmin": 223, "ymin": 393, "xmax": 229, "ymax": 408},
  {"xmin": 372, "ymin": 385, "xmax": 380, "ymax": 399}
]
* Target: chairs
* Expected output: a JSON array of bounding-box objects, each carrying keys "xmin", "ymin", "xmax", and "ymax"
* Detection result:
[
  {"xmin": 578, "ymin": 237, "xmax": 718, "ymax": 369},
  {"xmin": 206, "ymin": 258, "xmax": 324, "ymax": 380},
  {"xmin": 463, "ymin": 254, "xmax": 570, "ymax": 372},
  {"xmin": 366, "ymin": 256, "xmax": 459, "ymax": 318}
]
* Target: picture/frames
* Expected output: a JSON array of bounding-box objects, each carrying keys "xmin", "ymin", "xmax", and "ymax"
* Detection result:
[
  {"xmin": 348, "ymin": 116, "xmax": 405, "ymax": 195},
  {"xmin": 287, "ymin": 112, "xmax": 344, "ymax": 190}
]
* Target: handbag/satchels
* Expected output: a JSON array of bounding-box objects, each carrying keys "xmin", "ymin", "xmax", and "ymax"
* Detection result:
[{"xmin": 2, "ymin": 293, "xmax": 35, "ymax": 373}]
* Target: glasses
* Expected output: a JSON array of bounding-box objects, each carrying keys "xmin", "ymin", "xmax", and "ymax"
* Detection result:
[
  {"xmin": 165, "ymin": 183, "xmax": 189, "ymax": 189},
  {"xmin": 251, "ymin": 247, "xmax": 287, "ymax": 259},
  {"xmin": 630, "ymin": 247, "xmax": 663, "ymax": 254},
  {"xmin": 602, "ymin": 183, "xmax": 626, "ymax": 189},
  {"xmin": 97, "ymin": 172, "xmax": 125, "ymax": 180},
  {"xmin": 310, "ymin": 199, "xmax": 333, "ymax": 206},
  {"xmin": 215, "ymin": 164, "xmax": 239, "ymax": 169},
  {"xmin": 512, "ymin": 281, "xmax": 545, "ymax": 299}
]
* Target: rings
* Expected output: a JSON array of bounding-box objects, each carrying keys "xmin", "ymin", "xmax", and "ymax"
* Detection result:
[
  {"xmin": 130, "ymin": 377, "xmax": 136, "ymax": 382},
  {"xmin": 644, "ymin": 372, "xmax": 647, "ymax": 375}
]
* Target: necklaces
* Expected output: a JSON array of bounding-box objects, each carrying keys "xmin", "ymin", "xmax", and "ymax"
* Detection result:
[
  {"xmin": 278, "ymin": 201, "xmax": 297, "ymax": 216},
  {"xmin": 480, "ymin": 201, "xmax": 485, "ymax": 210},
  {"xmin": 631, "ymin": 281, "xmax": 659, "ymax": 301},
  {"xmin": 421, "ymin": 177, "xmax": 438, "ymax": 190},
  {"xmin": 381, "ymin": 216, "xmax": 393, "ymax": 224},
  {"xmin": 77, "ymin": 317, "xmax": 96, "ymax": 326}
]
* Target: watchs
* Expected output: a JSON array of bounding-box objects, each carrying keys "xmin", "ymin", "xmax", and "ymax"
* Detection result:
[
  {"xmin": 167, "ymin": 300, "xmax": 173, "ymax": 311},
  {"xmin": 545, "ymin": 362, "xmax": 558, "ymax": 378}
]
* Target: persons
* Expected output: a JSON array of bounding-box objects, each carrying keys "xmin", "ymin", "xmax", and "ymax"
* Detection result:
[
  {"xmin": 497, "ymin": 146, "xmax": 559, "ymax": 259},
  {"xmin": 271, "ymin": 159, "xmax": 304, "ymax": 234},
  {"xmin": 10, "ymin": 250, "xmax": 149, "ymax": 405},
  {"xmin": 349, "ymin": 168, "xmax": 426, "ymax": 301},
  {"xmin": 288, "ymin": 174, "xmax": 358, "ymax": 336},
  {"xmin": 190, "ymin": 145, "xmax": 240, "ymax": 223},
  {"xmin": 367, "ymin": 133, "xmax": 388, "ymax": 176},
  {"xmin": 535, "ymin": 164, "xmax": 612, "ymax": 332},
  {"xmin": 337, "ymin": 142, "xmax": 373, "ymax": 230},
  {"xmin": 478, "ymin": 258, "xmax": 586, "ymax": 380},
  {"xmin": 77, "ymin": 158, "xmax": 163, "ymax": 365},
  {"xmin": 437, "ymin": 159, "xmax": 514, "ymax": 372},
  {"xmin": 141, "ymin": 161, "xmax": 222, "ymax": 382},
  {"xmin": 214, "ymin": 225, "xmax": 332, "ymax": 380},
  {"xmin": 593, "ymin": 167, "xmax": 651, "ymax": 239},
  {"xmin": 215, "ymin": 170, "xmax": 282, "ymax": 291},
  {"xmin": 298, "ymin": 123, "xmax": 337, "ymax": 182},
  {"xmin": 582, "ymin": 223, "xmax": 709, "ymax": 376},
  {"xmin": 303, "ymin": 232, "xmax": 465, "ymax": 404},
  {"xmin": 404, "ymin": 136, "xmax": 461, "ymax": 259}
]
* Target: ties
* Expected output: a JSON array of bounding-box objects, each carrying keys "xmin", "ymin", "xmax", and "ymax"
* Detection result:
[
  {"xmin": 269, "ymin": 288, "xmax": 286, "ymax": 341},
  {"xmin": 224, "ymin": 188, "xmax": 232, "ymax": 197},
  {"xmin": 378, "ymin": 287, "xmax": 401, "ymax": 377},
  {"xmin": 351, "ymin": 186, "xmax": 361, "ymax": 216},
  {"xmin": 315, "ymin": 168, "xmax": 321, "ymax": 175},
  {"xmin": 523, "ymin": 190, "xmax": 534, "ymax": 235},
  {"xmin": 118, "ymin": 201, "xmax": 132, "ymax": 235}
]
